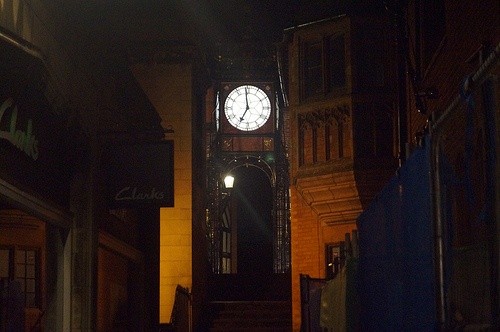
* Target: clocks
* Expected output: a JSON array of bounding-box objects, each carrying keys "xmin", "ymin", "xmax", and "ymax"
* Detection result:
[{"xmin": 224, "ymin": 85, "xmax": 271, "ymax": 132}]
